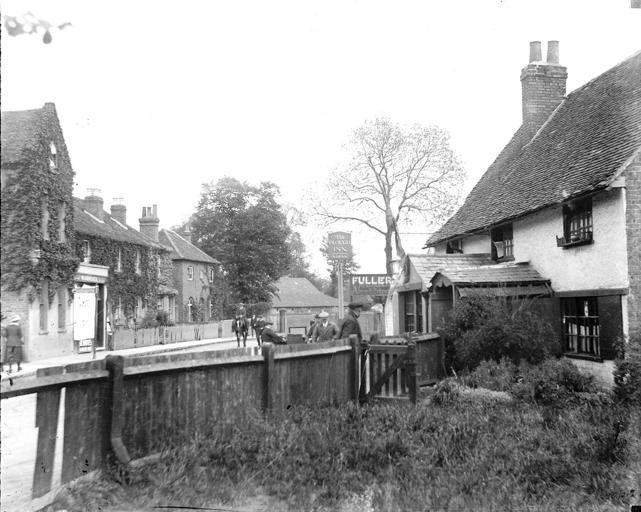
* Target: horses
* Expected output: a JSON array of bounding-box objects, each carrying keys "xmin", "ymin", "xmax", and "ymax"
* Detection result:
[{"xmin": 233, "ymin": 314, "xmax": 249, "ymax": 348}]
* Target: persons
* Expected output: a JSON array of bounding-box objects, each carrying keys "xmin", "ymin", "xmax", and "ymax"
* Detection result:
[
  {"xmin": 0, "ymin": 314, "xmax": 7, "ymax": 372},
  {"xmin": 3, "ymin": 315, "xmax": 24, "ymax": 373},
  {"xmin": 304, "ymin": 301, "xmax": 363, "ymax": 343},
  {"xmin": 235, "ymin": 302, "xmax": 287, "ymax": 345}
]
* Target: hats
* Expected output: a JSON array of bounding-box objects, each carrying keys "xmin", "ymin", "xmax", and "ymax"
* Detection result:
[
  {"xmin": 319, "ymin": 312, "xmax": 330, "ymax": 320},
  {"xmin": 348, "ymin": 301, "xmax": 363, "ymax": 310},
  {"xmin": 238, "ymin": 303, "xmax": 245, "ymax": 307}
]
